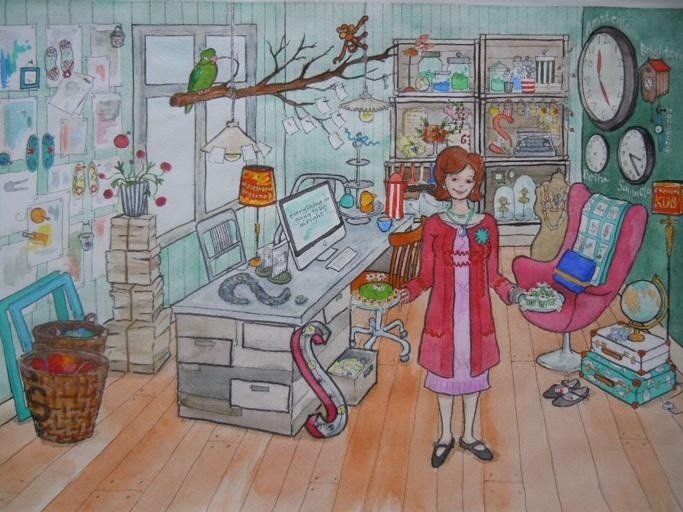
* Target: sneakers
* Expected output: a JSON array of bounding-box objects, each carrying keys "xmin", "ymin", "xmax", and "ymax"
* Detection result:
[
  {"xmin": 459, "ymin": 434, "xmax": 493, "ymax": 462},
  {"xmin": 541, "ymin": 376, "xmax": 583, "ymax": 399},
  {"xmin": 430, "ymin": 436, "xmax": 456, "ymax": 468},
  {"xmin": 552, "ymin": 386, "xmax": 591, "ymax": 408}
]
